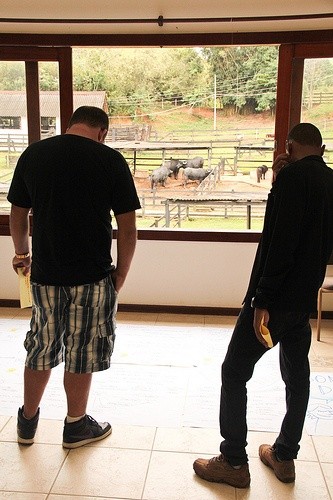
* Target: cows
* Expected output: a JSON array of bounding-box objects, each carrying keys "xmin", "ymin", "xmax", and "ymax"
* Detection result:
[
  {"xmin": 148, "ymin": 156, "xmax": 215, "ymax": 194},
  {"xmin": 256, "ymin": 165, "xmax": 268, "ymax": 183}
]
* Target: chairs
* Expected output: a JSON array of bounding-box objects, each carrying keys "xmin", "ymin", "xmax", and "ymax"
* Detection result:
[{"xmin": 316, "ymin": 276, "xmax": 333, "ymax": 341}]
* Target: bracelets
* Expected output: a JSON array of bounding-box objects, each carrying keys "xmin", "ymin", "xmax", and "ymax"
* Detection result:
[{"xmin": 15, "ymin": 252, "xmax": 30, "ymax": 259}]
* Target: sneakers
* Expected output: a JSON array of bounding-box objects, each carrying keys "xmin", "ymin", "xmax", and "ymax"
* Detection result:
[
  {"xmin": 62, "ymin": 413, "xmax": 112, "ymax": 449},
  {"xmin": 259, "ymin": 444, "xmax": 295, "ymax": 482},
  {"xmin": 16, "ymin": 405, "xmax": 41, "ymax": 443},
  {"xmin": 193, "ymin": 453, "xmax": 250, "ymax": 488}
]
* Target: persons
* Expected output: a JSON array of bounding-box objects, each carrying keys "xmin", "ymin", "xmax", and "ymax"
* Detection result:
[
  {"xmin": 192, "ymin": 123, "xmax": 333, "ymax": 489},
  {"xmin": 6, "ymin": 106, "xmax": 142, "ymax": 447}
]
